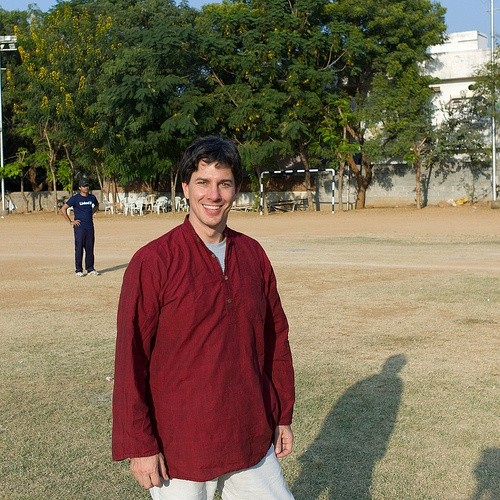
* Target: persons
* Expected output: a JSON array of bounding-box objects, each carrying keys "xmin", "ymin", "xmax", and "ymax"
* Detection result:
[
  {"xmin": 111, "ymin": 135, "xmax": 295, "ymax": 500},
  {"xmin": 60, "ymin": 179, "xmax": 100, "ymax": 277}
]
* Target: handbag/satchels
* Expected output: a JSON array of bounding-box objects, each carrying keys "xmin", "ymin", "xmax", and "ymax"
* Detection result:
[{"xmin": 8, "ymin": 200, "xmax": 18, "ymax": 211}]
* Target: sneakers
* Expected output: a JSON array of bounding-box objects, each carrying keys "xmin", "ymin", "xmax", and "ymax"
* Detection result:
[
  {"xmin": 76, "ymin": 272, "xmax": 84, "ymax": 277},
  {"xmin": 88, "ymin": 270, "xmax": 100, "ymax": 276}
]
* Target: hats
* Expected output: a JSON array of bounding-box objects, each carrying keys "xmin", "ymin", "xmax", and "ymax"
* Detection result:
[{"xmin": 79, "ymin": 178, "xmax": 90, "ymax": 187}]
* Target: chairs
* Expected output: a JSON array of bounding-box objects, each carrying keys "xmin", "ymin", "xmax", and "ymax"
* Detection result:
[{"xmin": 102, "ymin": 193, "xmax": 189, "ymax": 216}]
